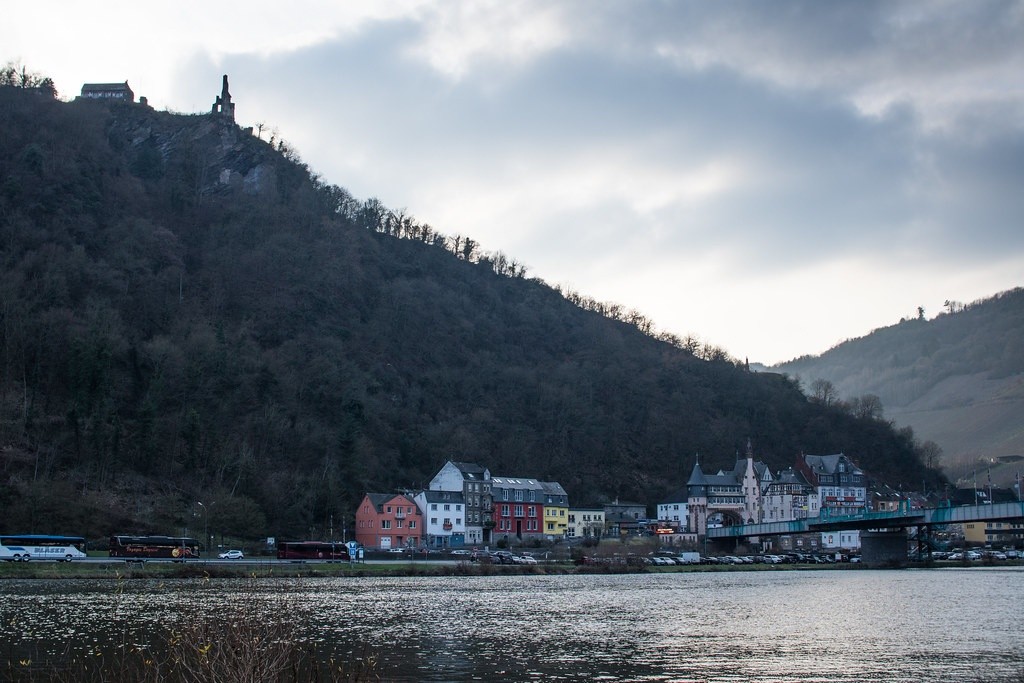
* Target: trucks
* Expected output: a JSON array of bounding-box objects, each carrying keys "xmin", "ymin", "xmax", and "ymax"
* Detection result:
[{"xmin": 677, "ymin": 552, "xmax": 700, "ymax": 565}]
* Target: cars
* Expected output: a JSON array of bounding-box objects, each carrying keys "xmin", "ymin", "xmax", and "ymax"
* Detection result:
[
  {"xmin": 640, "ymin": 557, "xmax": 652, "ymax": 564},
  {"xmin": 649, "ymin": 557, "xmax": 666, "ymax": 566},
  {"xmin": 700, "ymin": 556, "xmax": 764, "ymax": 565},
  {"xmin": 519, "ymin": 556, "xmax": 537, "ymax": 565},
  {"xmin": 931, "ymin": 551, "xmax": 1024, "ymax": 560},
  {"xmin": 217, "ymin": 550, "xmax": 244, "ymax": 560},
  {"xmin": 670, "ymin": 557, "xmax": 686, "ymax": 565},
  {"xmin": 659, "ymin": 557, "xmax": 676, "ymax": 566},
  {"xmin": 778, "ymin": 554, "xmax": 862, "ymax": 564},
  {"xmin": 763, "ymin": 555, "xmax": 782, "ymax": 564},
  {"xmin": 451, "ymin": 550, "xmax": 521, "ymax": 565}
]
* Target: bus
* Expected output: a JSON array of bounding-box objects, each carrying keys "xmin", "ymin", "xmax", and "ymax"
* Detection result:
[
  {"xmin": 0, "ymin": 535, "xmax": 90, "ymax": 562},
  {"xmin": 277, "ymin": 541, "xmax": 349, "ymax": 560},
  {"xmin": 109, "ymin": 536, "xmax": 204, "ymax": 563}
]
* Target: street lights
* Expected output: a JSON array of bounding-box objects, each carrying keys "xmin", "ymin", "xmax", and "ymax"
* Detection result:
[{"xmin": 198, "ymin": 502, "xmax": 207, "ymax": 563}]
